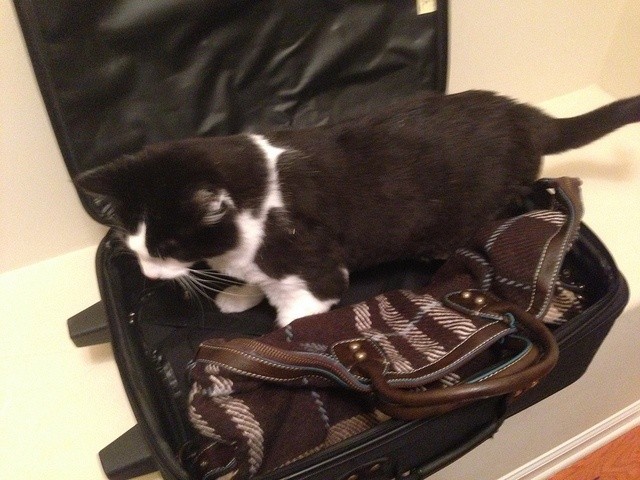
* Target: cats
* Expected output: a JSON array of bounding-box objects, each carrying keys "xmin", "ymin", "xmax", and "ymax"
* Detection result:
[{"xmin": 70, "ymin": 91, "xmax": 640, "ymax": 326}]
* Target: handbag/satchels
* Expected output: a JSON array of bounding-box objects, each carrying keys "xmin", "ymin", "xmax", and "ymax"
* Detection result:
[{"xmin": 176, "ymin": 176, "xmax": 592, "ymax": 480}]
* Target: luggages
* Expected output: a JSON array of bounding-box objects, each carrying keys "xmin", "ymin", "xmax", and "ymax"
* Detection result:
[{"xmin": 13, "ymin": 0, "xmax": 630, "ymax": 480}]
